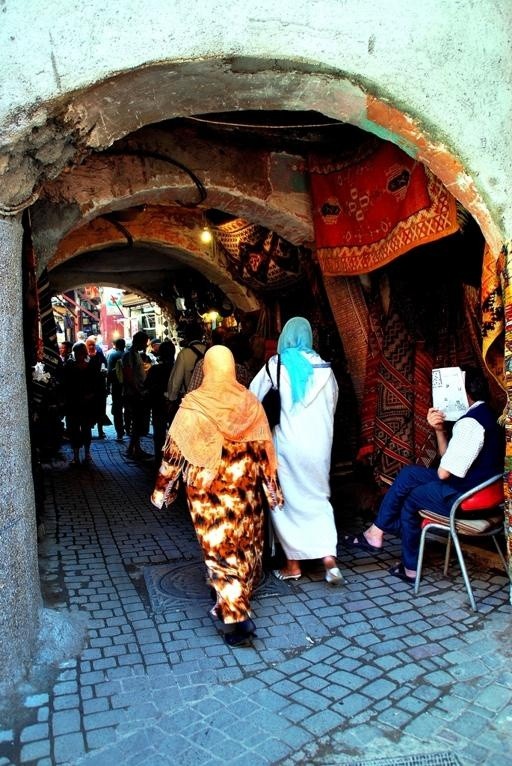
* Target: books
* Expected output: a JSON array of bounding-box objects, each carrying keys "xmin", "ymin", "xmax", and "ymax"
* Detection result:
[{"xmin": 430, "ymin": 367, "xmax": 469, "ymax": 423}]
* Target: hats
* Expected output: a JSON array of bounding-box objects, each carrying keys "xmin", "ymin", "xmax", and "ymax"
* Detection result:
[{"xmin": 151, "ymin": 339, "xmax": 162, "ymax": 345}]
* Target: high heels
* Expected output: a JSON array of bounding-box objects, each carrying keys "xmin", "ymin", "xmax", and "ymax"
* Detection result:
[
  {"xmin": 273, "ymin": 569, "xmax": 301, "ymax": 581},
  {"xmin": 325, "ymin": 568, "xmax": 345, "ymax": 585}
]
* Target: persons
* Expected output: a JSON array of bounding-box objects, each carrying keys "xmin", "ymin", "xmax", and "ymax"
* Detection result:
[
  {"xmin": 344, "ymin": 367, "xmax": 503, "ymax": 582},
  {"xmin": 248, "ymin": 317, "xmax": 342, "ymax": 584},
  {"xmin": 50, "ymin": 322, "xmax": 278, "ymax": 461},
  {"xmin": 150, "ymin": 342, "xmax": 283, "ymax": 645}
]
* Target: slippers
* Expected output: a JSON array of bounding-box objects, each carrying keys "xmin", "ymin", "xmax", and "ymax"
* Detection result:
[
  {"xmin": 389, "ymin": 562, "xmax": 422, "ymax": 583},
  {"xmin": 342, "ymin": 532, "xmax": 385, "ymax": 553}
]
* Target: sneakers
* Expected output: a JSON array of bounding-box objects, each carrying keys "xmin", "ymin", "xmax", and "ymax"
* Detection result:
[{"xmin": 122, "ymin": 450, "xmax": 155, "ymax": 463}]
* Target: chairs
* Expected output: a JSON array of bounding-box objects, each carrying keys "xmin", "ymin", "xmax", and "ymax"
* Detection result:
[{"xmin": 413, "ymin": 471, "xmax": 510, "ymax": 612}]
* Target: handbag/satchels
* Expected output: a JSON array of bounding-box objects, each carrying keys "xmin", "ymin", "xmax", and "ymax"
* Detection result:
[{"xmin": 261, "ymin": 354, "xmax": 280, "ymax": 431}]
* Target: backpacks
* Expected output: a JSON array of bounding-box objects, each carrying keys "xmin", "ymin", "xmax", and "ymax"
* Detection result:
[{"xmin": 185, "ymin": 344, "xmax": 211, "ymax": 394}]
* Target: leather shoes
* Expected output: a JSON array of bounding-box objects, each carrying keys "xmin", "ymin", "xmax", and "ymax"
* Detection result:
[{"xmin": 225, "ymin": 619, "xmax": 257, "ymax": 645}]
